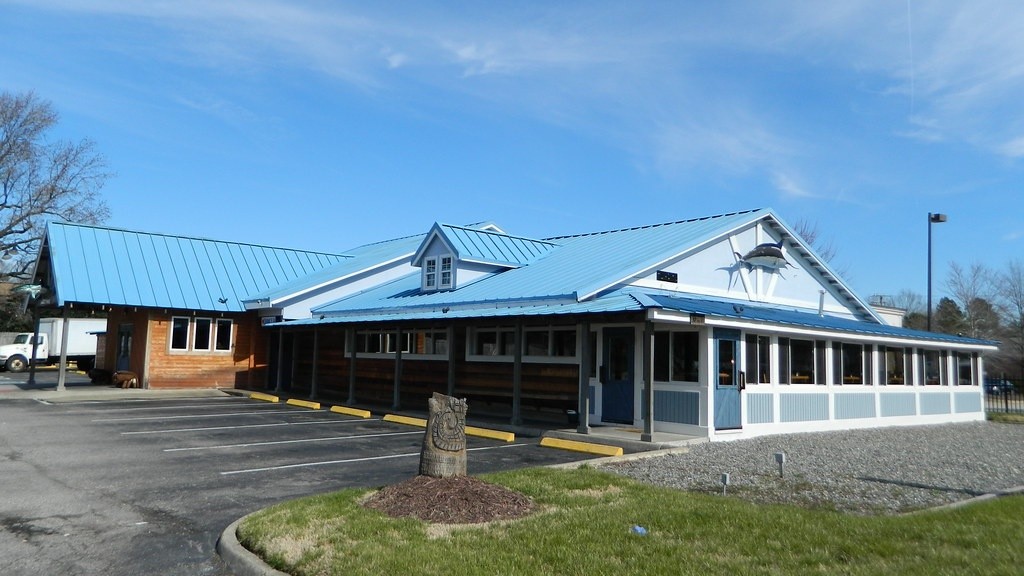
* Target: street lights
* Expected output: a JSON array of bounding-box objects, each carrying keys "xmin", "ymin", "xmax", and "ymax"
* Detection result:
[{"xmin": 928, "ymin": 211, "xmax": 947, "ymax": 331}]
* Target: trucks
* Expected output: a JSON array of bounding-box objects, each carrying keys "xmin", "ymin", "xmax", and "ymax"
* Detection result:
[{"xmin": 0, "ymin": 317, "xmax": 108, "ymax": 373}]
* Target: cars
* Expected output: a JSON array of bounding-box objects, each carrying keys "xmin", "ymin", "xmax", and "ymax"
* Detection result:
[{"xmin": 984, "ymin": 377, "xmax": 1015, "ymax": 396}]
